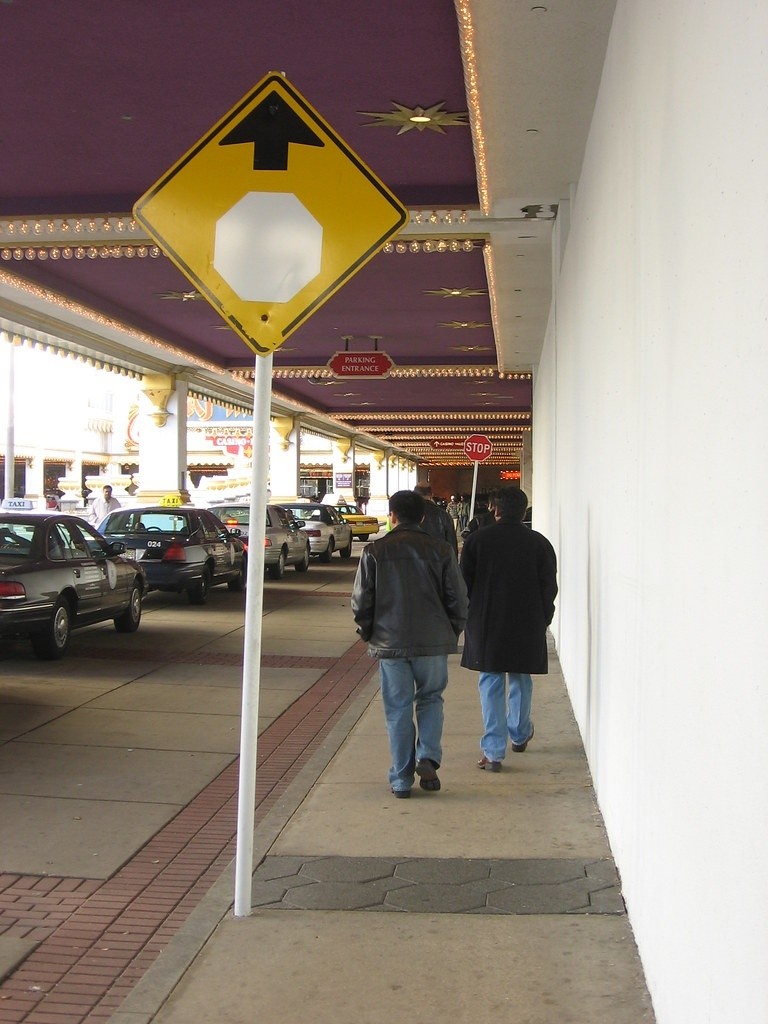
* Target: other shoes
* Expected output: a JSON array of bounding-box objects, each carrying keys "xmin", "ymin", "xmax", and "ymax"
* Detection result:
[
  {"xmin": 477, "ymin": 756, "xmax": 502, "ymax": 771},
  {"xmin": 511, "ymin": 720, "xmax": 535, "ymax": 753},
  {"xmin": 415, "ymin": 758, "xmax": 441, "ymax": 791},
  {"xmin": 391, "ymin": 786, "xmax": 410, "ymax": 797}
]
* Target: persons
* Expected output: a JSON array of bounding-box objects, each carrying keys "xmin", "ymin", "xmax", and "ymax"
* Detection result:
[
  {"xmin": 414, "ymin": 481, "xmax": 497, "ymax": 565},
  {"xmin": 89, "ymin": 485, "xmax": 121, "ymax": 530},
  {"xmin": 461, "ymin": 486, "xmax": 556, "ymax": 773},
  {"xmin": 352, "ymin": 490, "xmax": 466, "ymax": 798}
]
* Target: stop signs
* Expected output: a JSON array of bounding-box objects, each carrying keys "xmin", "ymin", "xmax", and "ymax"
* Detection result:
[{"xmin": 464, "ymin": 433, "xmax": 493, "ymax": 461}]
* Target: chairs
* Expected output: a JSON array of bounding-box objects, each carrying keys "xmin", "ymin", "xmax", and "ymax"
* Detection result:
[{"xmin": 222, "ymin": 514, "xmax": 232, "ymax": 523}]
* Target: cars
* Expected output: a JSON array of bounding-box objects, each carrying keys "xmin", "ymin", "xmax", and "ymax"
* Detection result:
[
  {"xmin": 312, "ymin": 497, "xmax": 380, "ymax": 543},
  {"xmin": 275, "ymin": 503, "xmax": 353, "ymax": 563},
  {"xmin": 0, "ymin": 496, "xmax": 151, "ymax": 661},
  {"xmin": 205, "ymin": 503, "xmax": 311, "ymax": 579},
  {"xmin": 88, "ymin": 493, "xmax": 249, "ymax": 606}
]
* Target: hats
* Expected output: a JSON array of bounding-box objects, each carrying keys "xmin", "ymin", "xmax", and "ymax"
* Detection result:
[{"xmin": 451, "ymin": 496, "xmax": 454, "ymax": 499}]
